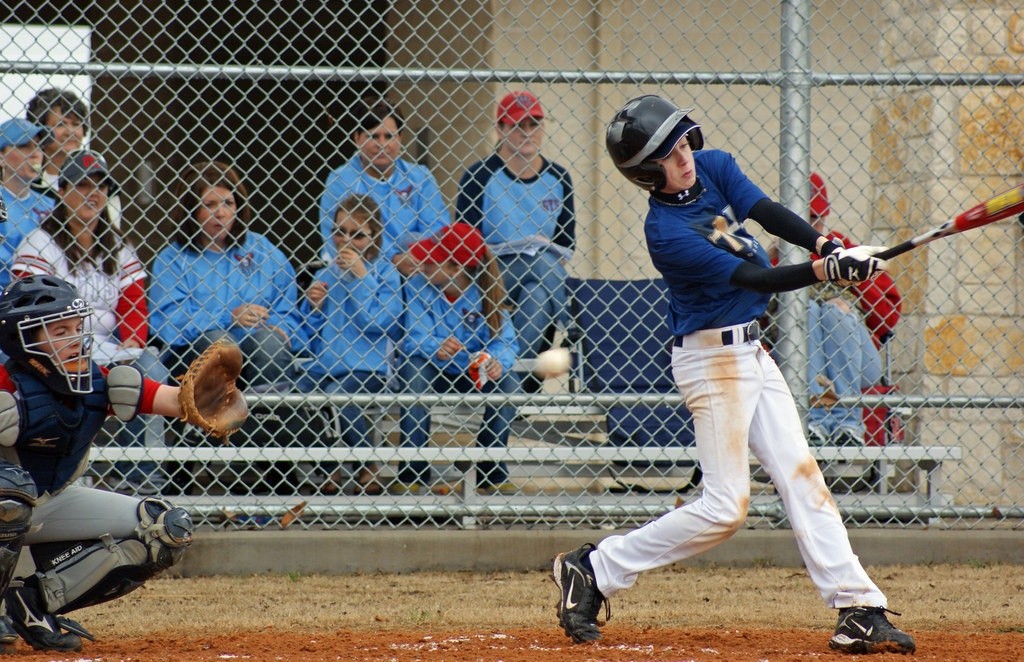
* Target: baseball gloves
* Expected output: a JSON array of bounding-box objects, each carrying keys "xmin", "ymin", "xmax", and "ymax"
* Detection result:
[{"xmin": 178, "ymin": 337, "xmax": 250, "ymax": 436}]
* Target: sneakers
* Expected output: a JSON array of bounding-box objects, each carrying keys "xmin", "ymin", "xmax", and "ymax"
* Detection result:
[
  {"xmin": 0, "ymin": 575, "xmax": 95, "ymax": 652},
  {"xmin": 550, "ymin": 543, "xmax": 610, "ymax": 644},
  {"xmin": 833, "ymin": 426, "xmax": 865, "ymax": 446},
  {"xmin": 807, "ymin": 422, "xmax": 830, "ymax": 446},
  {"xmin": 828, "ymin": 603, "xmax": 915, "ymax": 655}
]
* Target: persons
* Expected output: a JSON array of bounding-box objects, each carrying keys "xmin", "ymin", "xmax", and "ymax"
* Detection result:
[
  {"xmin": 147, "ymin": 162, "xmax": 297, "ymax": 494},
  {"xmin": 765, "ymin": 172, "xmax": 901, "ymax": 447},
  {"xmin": 551, "ymin": 94, "xmax": 917, "ymax": 655},
  {"xmin": 0, "ymin": 274, "xmax": 247, "ymax": 653},
  {"xmin": 292, "ymin": 193, "xmax": 403, "ymax": 493},
  {"xmin": 318, "ymin": 96, "xmax": 450, "ymax": 275},
  {"xmin": 394, "ymin": 223, "xmax": 526, "ymax": 495},
  {"xmin": 0, "ymin": 90, "xmax": 166, "ymax": 491},
  {"xmin": 454, "ymin": 91, "xmax": 585, "ymax": 416}
]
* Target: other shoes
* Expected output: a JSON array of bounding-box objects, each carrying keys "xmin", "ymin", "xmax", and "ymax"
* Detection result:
[
  {"xmin": 322, "ymin": 472, "xmax": 343, "ymax": 494},
  {"xmin": 390, "ymin": 480, "xmax": 424, "ymax": 495},
  {"xmin": 485, "ymin": 480, "xmax": 518, "ymax": 495},
  {"xmin": 356, "ymin": 466, "xmax": 383, "ymax": 494}
]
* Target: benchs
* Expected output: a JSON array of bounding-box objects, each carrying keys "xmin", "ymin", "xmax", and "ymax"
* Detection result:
[{"xmin": 89, "ymin": 358, "xmax": 963, "ymax": 528}]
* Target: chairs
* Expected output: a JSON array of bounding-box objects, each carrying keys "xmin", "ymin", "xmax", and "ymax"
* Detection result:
[{"xmin": 560, "ymin": 276, "xmax": 706, "ymax": 493}]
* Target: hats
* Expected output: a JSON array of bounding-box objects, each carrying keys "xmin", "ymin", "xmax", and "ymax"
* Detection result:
[
  {"xmin": 408, "ymin": 223, "xmax": 487, "ymax": 267},
  {"xmin": 0, "ymin": 117, "xmax": 55, "ymax": 149},
  {"xmin": 496, "ymin": 91, "xmax": 545, "ymax": 125},
  {"xmin": 58, "ymin": 149, "xmax": 113, "ymax": 194},
  {"xmin": 807, "ymin": 175, "xmax": 830, "ymax": 215}
]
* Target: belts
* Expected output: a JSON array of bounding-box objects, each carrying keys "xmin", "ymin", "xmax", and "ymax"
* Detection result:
[{"xmin": 672, "ymin": 320, "xmax": 761, "ymax": 349}]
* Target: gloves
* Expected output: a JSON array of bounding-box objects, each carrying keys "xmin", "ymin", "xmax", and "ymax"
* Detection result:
[
  {"xmin": 823, "ymin": 246, "xmax": 890, "ymax": 283},
  {"xmin": 818, "ymin": 238, "xmax": 850, "ymax": 273}
]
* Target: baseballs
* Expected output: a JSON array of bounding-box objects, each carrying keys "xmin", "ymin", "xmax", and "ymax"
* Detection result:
[{"xmin": 534, "ymin": 347, "xmax": 572, "ymax": 377}]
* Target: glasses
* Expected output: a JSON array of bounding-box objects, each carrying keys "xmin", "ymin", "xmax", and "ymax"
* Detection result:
[{"xmin": 331, "ymin": 227, "xmax": 372, "ymax": 240}]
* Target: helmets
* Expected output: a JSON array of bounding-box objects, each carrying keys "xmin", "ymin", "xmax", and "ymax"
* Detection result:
[
  {"xmin": 604, "ymin": 94, "xmax": 704, "ymax": 192},
  {"xmin": 0, "ymin": 275, "xmax": 95, "ymax": 398}
]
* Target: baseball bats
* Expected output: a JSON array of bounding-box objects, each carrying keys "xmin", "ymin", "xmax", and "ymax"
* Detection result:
[{"xmin": 871, "ymin": 184, "xmax": 1024, "ymax": 262}]
